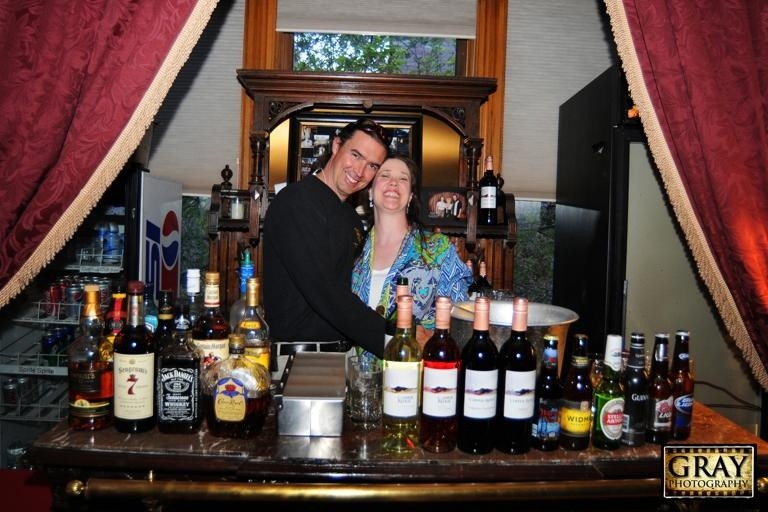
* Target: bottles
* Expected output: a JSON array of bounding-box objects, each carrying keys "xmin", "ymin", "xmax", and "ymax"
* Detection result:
[
  {"xmin": 64, "ymin": 244, "xmax": 274, "ymax": 437},
  {"xmin": 380, "ymin": 269, "xmax": 696, "ymax": 453},
  {"xmin": 79, "ymin": 218, "xmax": 124, "ymax": 274},
  {"xmin": 479, "ymin": 154, "xmax": 500, "ymax": 224}
]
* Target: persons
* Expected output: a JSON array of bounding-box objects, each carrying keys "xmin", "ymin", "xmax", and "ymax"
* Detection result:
[
  {"xmin": 264, "ymin": 119, "xmax": 435, "ymax": 370},
  {"xmin": 436, "ymin": 194, "xmax": 462, "ymax": 218},
  {"xmin": 351, "ymin": 155, "xmax": 476, "ymax": 373}
]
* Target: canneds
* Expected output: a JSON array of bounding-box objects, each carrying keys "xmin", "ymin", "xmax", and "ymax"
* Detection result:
[
  {"xmin": 94, "ymin": 223, "xmax": 124, "ymax": 265},
  {"xmin": 41, "ymin": 325, "xmax": 76, "ymax": 365},
  {"xmin": 45, "ymin": 272, "xmax": 112, "ymax": 321},
  {"xmin": 3, "ymin": 375, "xmax": 31, "ymax": 411}
]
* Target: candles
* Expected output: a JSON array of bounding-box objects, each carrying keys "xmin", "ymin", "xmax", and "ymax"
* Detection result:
[{"xmin": 230, "ymin": 201, "xmax": 244, "ymax": 220}]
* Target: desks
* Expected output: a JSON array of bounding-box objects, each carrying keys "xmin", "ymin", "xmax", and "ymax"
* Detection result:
[{"xmin": 26, "ymin": 398, "xmax": 768, "ymax": 511}]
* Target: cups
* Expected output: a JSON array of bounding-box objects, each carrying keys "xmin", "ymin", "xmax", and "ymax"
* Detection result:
[{"xmin": 347, "ymin": 351, "xmax": 385, "ymax": 424}]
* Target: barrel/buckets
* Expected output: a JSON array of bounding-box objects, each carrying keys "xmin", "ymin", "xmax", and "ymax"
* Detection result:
[{"xmin": 449, "ymin": 297, "xmax": 580, "ymax": 385}]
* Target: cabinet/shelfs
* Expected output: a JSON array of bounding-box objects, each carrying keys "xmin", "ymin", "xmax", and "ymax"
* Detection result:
[{"xmin": 2, "ymin": 232, "xmax": 121, "ymax": 425}]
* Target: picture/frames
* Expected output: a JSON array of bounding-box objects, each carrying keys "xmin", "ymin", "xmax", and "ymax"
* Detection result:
[{"xmin": 288, "ymin": 117, "xmax": 425, "ymax": 219}]
